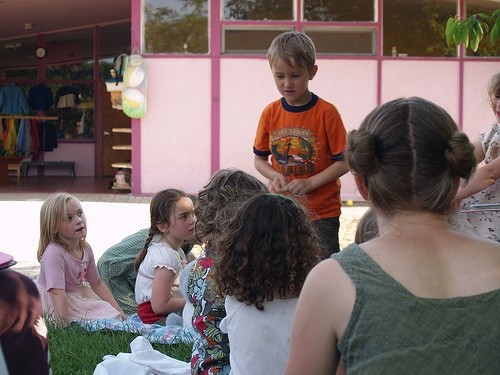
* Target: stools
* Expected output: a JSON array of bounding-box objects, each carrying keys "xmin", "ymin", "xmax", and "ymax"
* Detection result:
[{"xmin": 8, "ymin": 167, "xmax": 21, "ymax": 178}]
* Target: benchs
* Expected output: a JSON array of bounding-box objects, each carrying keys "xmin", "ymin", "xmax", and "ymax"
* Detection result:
[{"xmin": 22, "ymin": 161, "xmax": 76, "ymax": 177}]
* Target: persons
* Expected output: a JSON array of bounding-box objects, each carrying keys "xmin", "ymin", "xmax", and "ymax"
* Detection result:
[
  {"xmin": 445, "ymin": 73, "xmax": 500, "ymax": 242},
  {"xmin": 286, "ymin": 96, "xmax": 500, "ymax": 375},
  {"xmin": 0, "ymin": 169, "xmax": 500, "ymax": 375},
  {"xmin": 254, "ymin": 33, "xmax": 349, "ymax": 258}
]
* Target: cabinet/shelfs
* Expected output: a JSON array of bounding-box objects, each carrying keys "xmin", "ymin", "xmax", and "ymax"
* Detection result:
[{"xmin": 111, "ymin": 128, "xmax": 132, "ymax": 189}]
